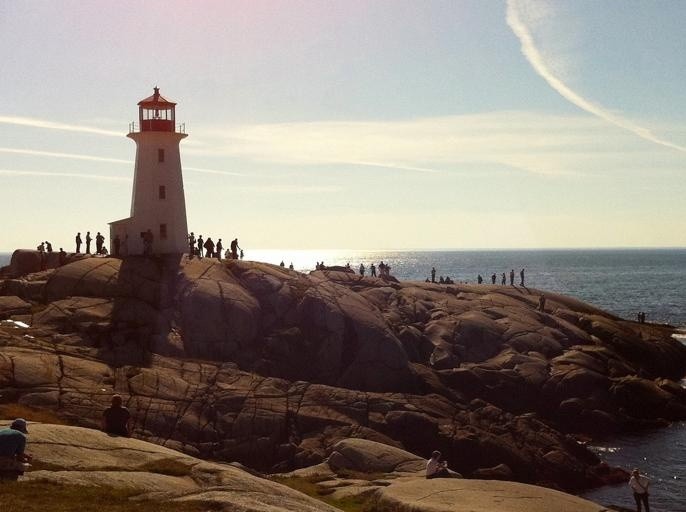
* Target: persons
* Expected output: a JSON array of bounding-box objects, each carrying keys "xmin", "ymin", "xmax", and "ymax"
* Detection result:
[
  {"xmin": 539, "ymin": 293, "xmax": 546, "ymax": 313},
  {"xmin": 280, "ymin": 260, "xmax": 284, "ymax": 266},
  {"xmin": 345, "ymin": 260, "xmax": 391, "ymax": 277},
  {"xmin": 76, "ymin": 232, "xmax": 121, "ymax": 257},
  {"xmin": 320, "ymin": 261, "xmax": 325, "ymax": 268},
  {"xmin": 0, "ymin": 421, "xmax": 32, "ymax": 475},
  {"xmin": 184, "ymin": 232, "xmax": 243, "ymax": 260},
  {"xmin": 316, "ymin": 262, "xmax": 321, "ymax": 270},
  {"xmin": 426, "ymin": 451, "xmax": 452, "ymax": 479},
  {"xmin": 425, "ymin": 266, "xmax": 525, "ymax": 285},
  {"xmin": 628, "ymin": 468, "xmax": 652, "ymax": 510},
  {"xmin": 100, "ymin": 395, "xmax": 130, "ymax": 438},
  {"xmin": 37, "ymin": 241, "xmax": 65, "ymax": 253},
  {"xmin": 290, "ymin": 262, "xmax": 294, "ymax": 269}
]
